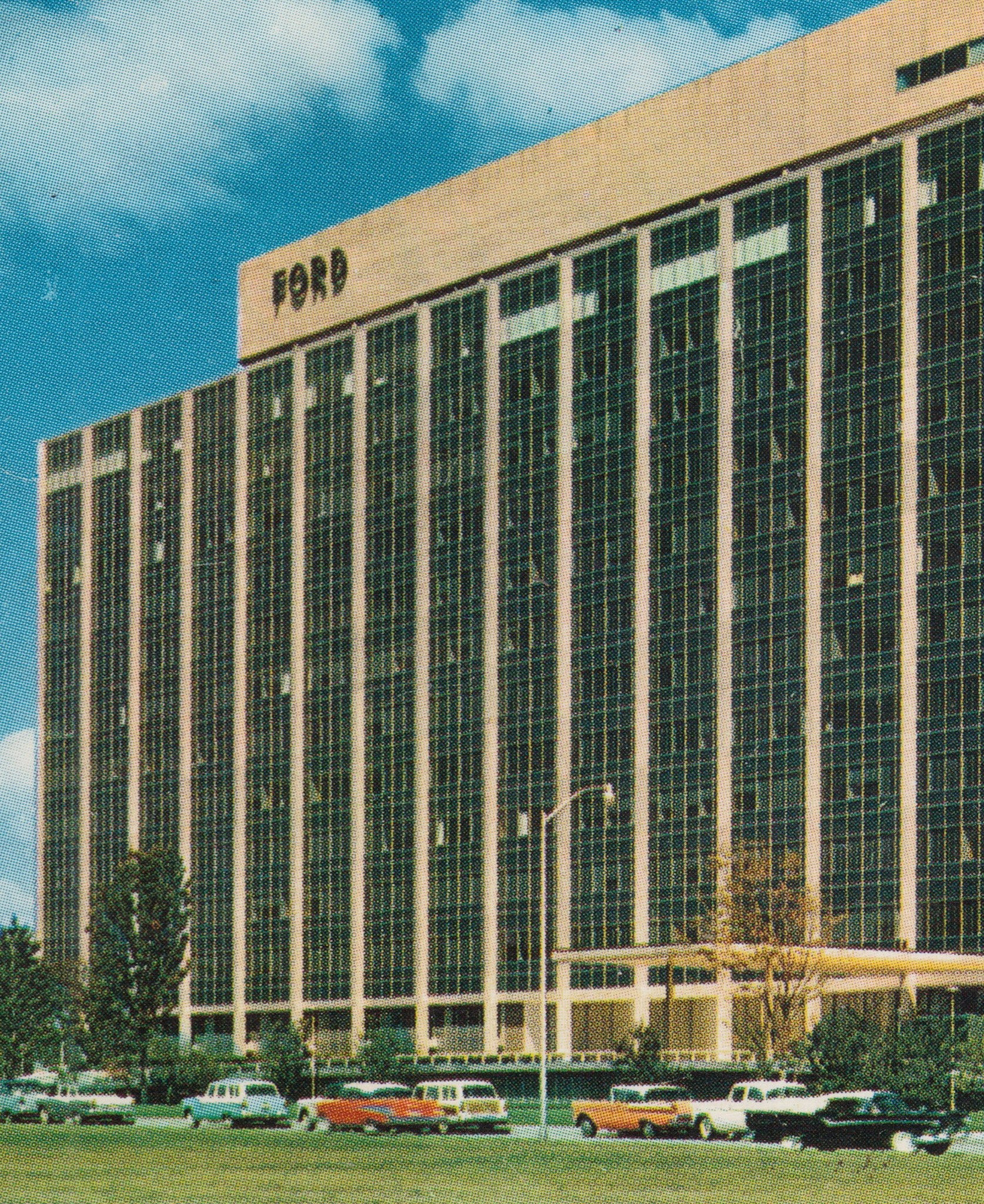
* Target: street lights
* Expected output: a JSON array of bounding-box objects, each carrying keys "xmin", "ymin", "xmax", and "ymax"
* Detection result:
[{"xmin": 540, "ymin": 782, "xmax": 617, "ymax": 1136}]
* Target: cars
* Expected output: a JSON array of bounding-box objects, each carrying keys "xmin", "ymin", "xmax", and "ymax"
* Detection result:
[
  {"xmin": 295, "ymin": 1081, "xmax": 346, "ymax": 1132},
  {"xmin": 0, "ymin": 1068, "xmax": 58, "ymax": 1126},
  {"xmin": 318, "ymin": 1078, "xmax": 443, "ymax": 1136},
  {"xmin": 411, "ymin": 1080, "xmax": 512, "ymax": 1135},
  {"xmin": 36, "ymin": 1071, "xmax": 136, "ymax": 1126},
  {"xmin": 181, "ymin": 1079, "xmax": 289, "ymax": 1131}
]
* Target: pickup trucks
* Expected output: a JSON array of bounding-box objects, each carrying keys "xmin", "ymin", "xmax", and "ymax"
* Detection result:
[
  {"xmin": 693, "ymin": 1080, "xmax": 812, "ymax": 1143},
  {"xmin": 743, "ymin": 1089, "xmax": 968, "ymax": 1155},
  {"xmin": 572, "ymin": 1084, "xmax": 696, "ymax": 1141}
]
